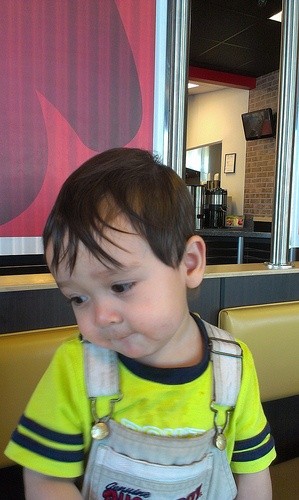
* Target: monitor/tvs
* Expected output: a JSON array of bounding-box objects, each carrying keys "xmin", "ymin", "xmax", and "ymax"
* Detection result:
[{"xmin": 241, "ymin": 107, "xmax": 275, "ymax": 141}]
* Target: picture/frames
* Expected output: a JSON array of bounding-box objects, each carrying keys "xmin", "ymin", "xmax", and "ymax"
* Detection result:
[{"xmin": 224, "ymin": 153, "xmax": 236, "ymax": 174}]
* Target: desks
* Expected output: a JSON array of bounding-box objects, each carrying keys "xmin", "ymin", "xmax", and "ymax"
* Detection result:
[{"xmin": 197, "ymin": 228, "xmax": 297, "ymax": 265}]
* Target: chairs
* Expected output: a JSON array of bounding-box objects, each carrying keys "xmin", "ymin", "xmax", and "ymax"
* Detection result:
[{"xmin": 0, "ymin": 300, "xmax": 299, "ymax": 500}]
